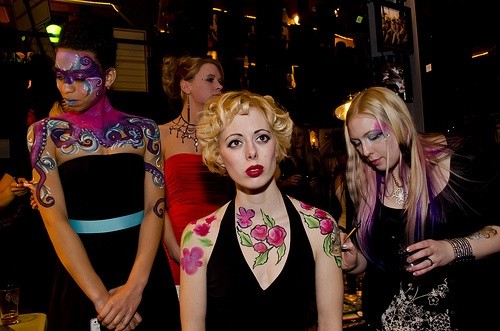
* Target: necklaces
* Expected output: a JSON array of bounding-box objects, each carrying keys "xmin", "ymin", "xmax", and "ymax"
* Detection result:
[
  {"xmin": 169, "ymin": 115, "xmax": 199, "ymax": 153},
  {"xmin": 390, "ymin": 169, "xmax": 410, "ymax": 205}
]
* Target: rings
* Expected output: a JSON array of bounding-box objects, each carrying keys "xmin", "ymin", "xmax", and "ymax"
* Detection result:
[
  {"xmin": 427, "ymin": 258, "xmax": 433, "ymax": 266},
  {"xmin": 16, "ymin": 183, "xmax": 19, "ymax": 188}
]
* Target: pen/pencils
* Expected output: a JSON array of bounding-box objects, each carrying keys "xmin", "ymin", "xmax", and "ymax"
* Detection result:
[{"xmin": 14, "ymin": 177, "xmax": 19, "ymax": 184}]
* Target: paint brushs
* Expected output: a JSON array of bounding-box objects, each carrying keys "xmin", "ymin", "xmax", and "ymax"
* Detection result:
[{"xmin": 344, "ymin": 227, "xmax": 357, "ymax": 243}]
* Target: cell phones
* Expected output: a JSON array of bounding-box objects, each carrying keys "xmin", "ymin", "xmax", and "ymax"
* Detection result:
[{"xmin": 90, "ymin": 319, "xmax": 109, "ymax": 331}]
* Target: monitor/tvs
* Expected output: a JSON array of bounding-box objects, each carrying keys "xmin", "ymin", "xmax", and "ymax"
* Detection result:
[{"xmin": 374, "ymin": 0, "xmax": 415, "ymax": 54}]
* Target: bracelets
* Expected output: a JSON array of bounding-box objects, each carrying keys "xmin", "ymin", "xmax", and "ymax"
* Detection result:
[{"xmin": 443, "ymin": 237, "xmax": 476, "ymax": 267}]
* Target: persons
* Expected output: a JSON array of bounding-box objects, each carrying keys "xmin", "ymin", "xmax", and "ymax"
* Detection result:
[
  {"xmin": 0, "ymin": 55, "xmax": 345, "ymax": 331},
  {"xmin": 382, "ymin": 14, "xmax": 407, "ymax": 44},
  {"xmin": 27, "ymin": 16, "xmax": 180, "ymax": 331},
  {"xmin": 340, "ymin": 87, "xmax": 500, "ymax": 331}
]
